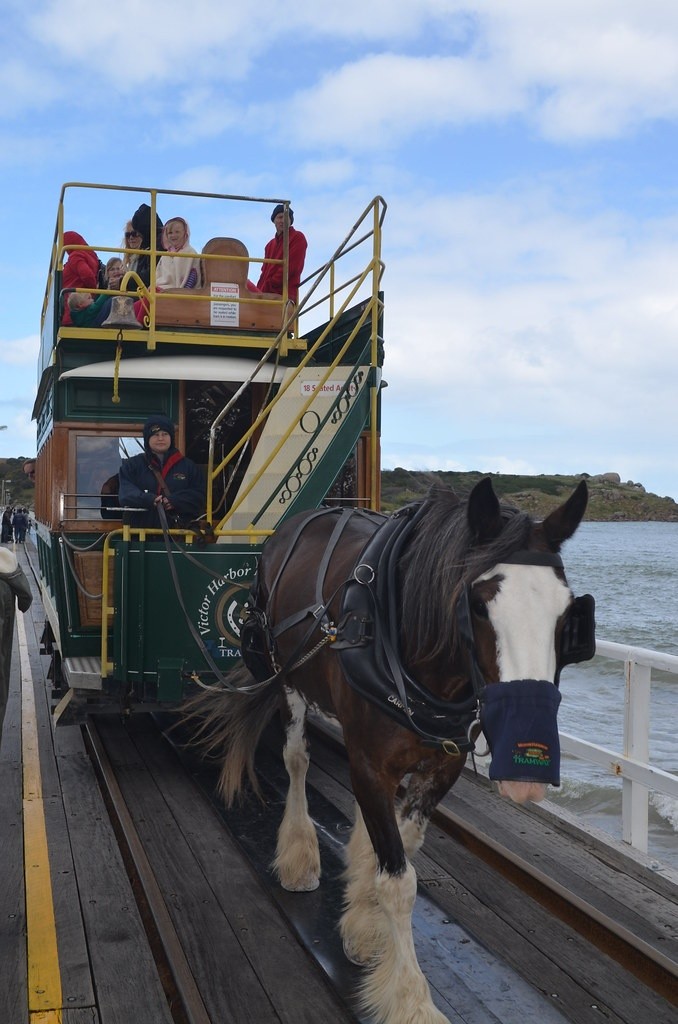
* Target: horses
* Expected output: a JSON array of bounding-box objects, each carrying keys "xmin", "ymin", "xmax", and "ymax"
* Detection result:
[{"xmin": 161, "ymin": 476, "xmax": 597, "ymax": 1024}]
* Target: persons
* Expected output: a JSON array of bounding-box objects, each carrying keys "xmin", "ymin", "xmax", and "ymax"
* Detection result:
[
  {"xmin": 55, "ymin": 203, "xmax": 203, "ymax": 328},
  {"xmin": 0, "ymin": 504, "xmax": 30, "ymax": 544},
  {"xmin": 22, "ymin": 458, "xmax": 36, "ymax": 483},
  {"xmin": 255, "ymin": 203, "xmax": 308, "ymax": 308},
  {"xmin": 118, "ymin": 415, "xmax": 205, "ymax": 540}
]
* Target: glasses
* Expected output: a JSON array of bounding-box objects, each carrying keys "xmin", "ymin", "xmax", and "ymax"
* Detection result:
[{"xmin": 125, "ymin": 231, "xmax": 138, "ymax": 238}]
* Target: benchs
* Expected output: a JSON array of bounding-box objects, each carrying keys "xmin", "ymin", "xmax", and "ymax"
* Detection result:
[{"xmin": 155, "ymin": 237, "xmax": 295, "ymax": 333}]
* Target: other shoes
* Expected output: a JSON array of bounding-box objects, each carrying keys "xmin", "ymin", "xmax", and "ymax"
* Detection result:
[
  {"xmin": 20, "ymin": 540, "xmax": 24, "ymax": 544},
  {"xmin": 15, "ymin": 539, "xmax": 18, "ymax": 544}
]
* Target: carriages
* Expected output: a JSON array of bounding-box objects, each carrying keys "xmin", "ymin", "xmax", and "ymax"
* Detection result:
[{"xmin": 24, "ymin": 178, "xmax": 602, "ymax": 1022}]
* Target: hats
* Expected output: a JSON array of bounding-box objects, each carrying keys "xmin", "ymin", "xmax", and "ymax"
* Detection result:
[
  {"xmin": 271, "ymin": 205, "xmax": 293, "ymax": 224},
  {"xmin": 143, "ymin": 415, "xmax": 175, "ymax": 449}
]
